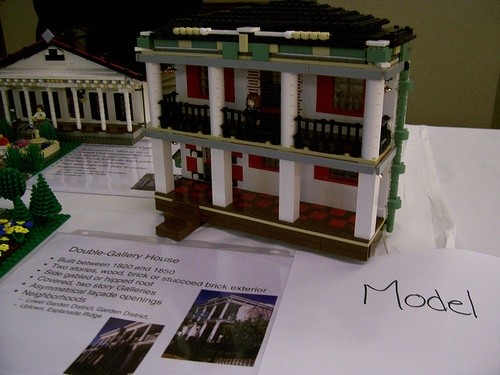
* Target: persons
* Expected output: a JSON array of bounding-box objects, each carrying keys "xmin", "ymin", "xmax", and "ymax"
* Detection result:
[
  {"xmin": 33, "ymin": 104, "xmax": 46, "ymax": 123},
  {"xmin": 241, "ymin": 92, "xmax": 262, "ymax": 138}
]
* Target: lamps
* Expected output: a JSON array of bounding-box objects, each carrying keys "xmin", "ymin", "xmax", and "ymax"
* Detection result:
[
  {"xmin": 384, "ymin": 79, "xmax": 393, "ymax": 93},
  {"xmin": 164, "ymin": 64, "xmax": 177, "ymax": 74}
]
